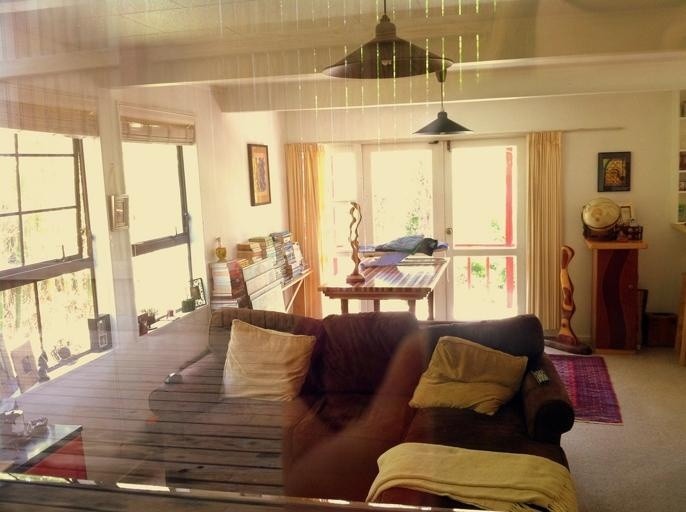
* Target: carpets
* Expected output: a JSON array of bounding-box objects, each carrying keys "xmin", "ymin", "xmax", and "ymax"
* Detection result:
[{"xmin": 543, "ymin": 351, "xmax": 623, "ymax": 426}]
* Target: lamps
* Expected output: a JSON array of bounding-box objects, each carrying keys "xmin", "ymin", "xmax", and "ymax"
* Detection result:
[{"xmin": 315, "ymin": 0, "xmax": 474, "ymax": 136}]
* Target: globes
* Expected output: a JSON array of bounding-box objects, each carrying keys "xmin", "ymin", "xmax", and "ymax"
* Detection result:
[{"xmin": 581, "ymin": 197, "xmax": 621, "ymax": 242}]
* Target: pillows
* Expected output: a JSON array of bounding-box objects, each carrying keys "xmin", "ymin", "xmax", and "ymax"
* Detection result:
[
  {"xmin": 408, "ymin": 334, "xmax": 532, "ymax": 419},
  {"xmin": 217, "ymin": 320, "xmax": 317, "ymax": 403}
]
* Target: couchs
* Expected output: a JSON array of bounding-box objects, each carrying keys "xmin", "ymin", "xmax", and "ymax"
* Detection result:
[{"xmin": 143, "ymin": 305, "xmax": 573, "ymax": 506}]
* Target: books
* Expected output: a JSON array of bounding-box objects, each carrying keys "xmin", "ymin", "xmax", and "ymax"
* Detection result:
[{"xmin": 208, "ymin": 232, "xmax": 304, "ymax": 310}]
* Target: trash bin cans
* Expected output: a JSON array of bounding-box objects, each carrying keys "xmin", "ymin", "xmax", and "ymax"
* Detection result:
[{"xmin": 645, "ymin": 312, "xmax": 677, "ymax": 347}]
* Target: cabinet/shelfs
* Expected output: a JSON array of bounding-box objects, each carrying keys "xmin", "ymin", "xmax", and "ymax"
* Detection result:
[{"xmin": 585, "ymin": 238, "xmax": 648, "ymax": 355}]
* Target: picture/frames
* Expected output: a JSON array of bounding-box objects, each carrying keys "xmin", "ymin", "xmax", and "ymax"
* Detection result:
[
  {"xmin": 248, "ymin": 142, "xmax": 273, "ymax": 206},
  {"xmin": 597, "ymin": 151, "xmax": 633, "ymax": 192},
  {"xmin": 318, "ymin": 255, "xmax": 451, "ymax": 323},
  {"xmin": 112, "ymin": 194, "xmax": 130, "ymax": 232}
]
